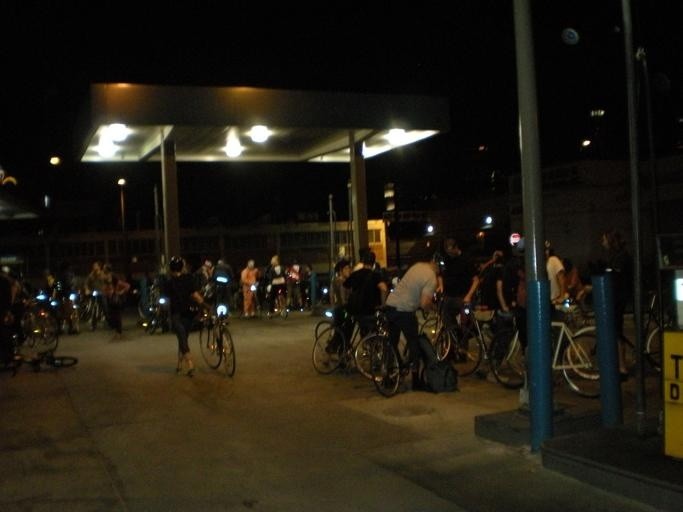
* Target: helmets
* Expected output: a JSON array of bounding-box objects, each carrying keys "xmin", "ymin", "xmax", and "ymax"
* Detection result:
[{"xmin": 168, "ymin": 255, "xmax": 183, "ymax": 268}]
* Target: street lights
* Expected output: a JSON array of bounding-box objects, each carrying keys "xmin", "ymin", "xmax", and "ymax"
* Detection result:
[
  {"xmin": 42, "ymin": 154, "xmax": 61, "ymax": 274},
  {"xmin": 384, "ymin": 188, "xmax": 402, "ymax": 275},
  {"xmin": 117, "ymin": 176, "xmax": 135, "ymax": 284}
]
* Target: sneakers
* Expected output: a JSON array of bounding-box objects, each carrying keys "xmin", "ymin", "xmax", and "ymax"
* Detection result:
[
  {"xmin": 175, "ymin": 357, "xmax": 182, "ymax": 372},
  {"xmin": 187, "ymin": 361, "xmax": 195, "ymax": 376},
  {"xmin": 322, "ymin": 350, "xmax": 331, "ymax": 366}
]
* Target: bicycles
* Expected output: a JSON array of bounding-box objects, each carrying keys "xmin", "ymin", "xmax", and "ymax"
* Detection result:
[
  {"xmin": 267, "ymin": 286, "xmax": 288, "ymax": 319},
  {"xmin": 5, "ymin": 290, "xmax": 106, "ymax": 352},
  {"xmin": 490, "ymin": 309, "xmax": 606, "ymax": 398},
  {"xmin": 371, "ymin": 301, "xmax": 451, "ymax": 397},
  {"xmin": 556, "ymin": 301, "xmax": 667, "ymax": 379},
  {"xmin": 150, "ymin": 297, "xmax": 166, "ymax": 334},
  {"xmin": 419, "ymin": 292, "xmax": 483, "ymax": 376},
  {"xmin": 312, "ymin": 305, "xmax": 397, "ymax": 379},
  {"xmin": 12, "ymin": 354, "xmax": 77, "ymax": 376},
  {"xmin": 189, "ymin": 303, "xmax": 235, "ymax": 377}
]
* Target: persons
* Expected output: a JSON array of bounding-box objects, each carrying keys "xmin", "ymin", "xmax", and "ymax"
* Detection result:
[
  {"xmin": 266, "ymin": 255, "xmax": 289, "ymax": 319},
  {"xmin": 321, "ymin": 258, "xmax": 352, "ymax": 368},
  {"xmin": 440, "ymin": 239, "xmax": 480, "ymax": 360},
  {"xmin": 163, "ymin": 256, "xmax": 204, "ymax": 376},
  {"xmin": 543, "ymin": 240, "xmax": 568, "ymax": 319},
  {"xmin": 211, "ymin": 256, "xmax": 236, "ymax": 308},
  {"xmin": 339, "ymin": 251, "xmax": 388, "ymax": 364},
  {"xmin": 382, "ymin": 243, "xmax": 438, "ymax": 391},
  {"xmin": 353, "ymin": 246, "xmax": 371, "ymax": 271},
  {"xmin": 87, "ymin": 262, "xmax": 103, "ymax": 298},
  {"xmin": 285, "ymin": 260, "xmax": 305, "ymax": 309},
  {"xmin": 494, "ymin": 247, "xmax": 526, "ymax": 378},
  {"xmin": 468, "ymin": 228, "xmax": 503, "ymax": 309},
  {"xmin": 302, "ymin": 262, "xmax": 320, "ymax": 311},
  {"xmin": 240, "ymin": 260, "xmax": 262, "ymax": 318},
  {"xmin": 95, "ymin": 264, "xmax": 132, "ymax": 338},
  {"xmin": 599, "ymin": 231, "xmax": 639, "ymax": 375}
]
